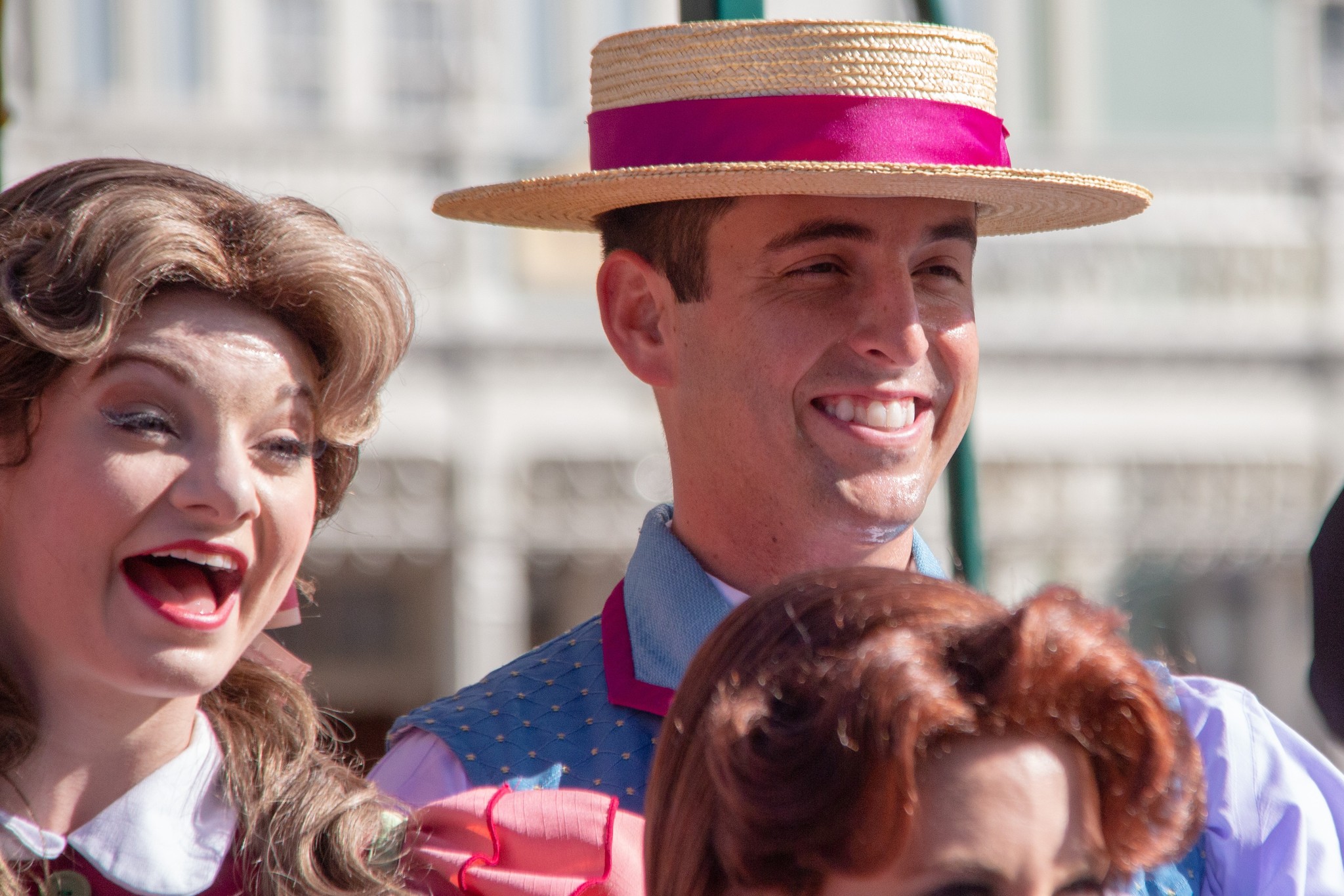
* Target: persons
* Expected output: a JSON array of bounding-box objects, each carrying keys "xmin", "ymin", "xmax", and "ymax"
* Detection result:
[
  {"xmin": 361, "ymin": 22, "xmax": 1344, "ymax": 896},
  {"xmin": 642, "ymin": 568, "xmax": 1206, "ymax": 896},
  {"xmin": 0, "ymin": 158, "xmax": 407, "ymax": 896}
]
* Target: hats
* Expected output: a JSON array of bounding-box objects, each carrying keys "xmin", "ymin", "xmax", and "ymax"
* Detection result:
[{"xmin": 433, "ymin": 19, "xmax": 1150, "ymax": 236}]
